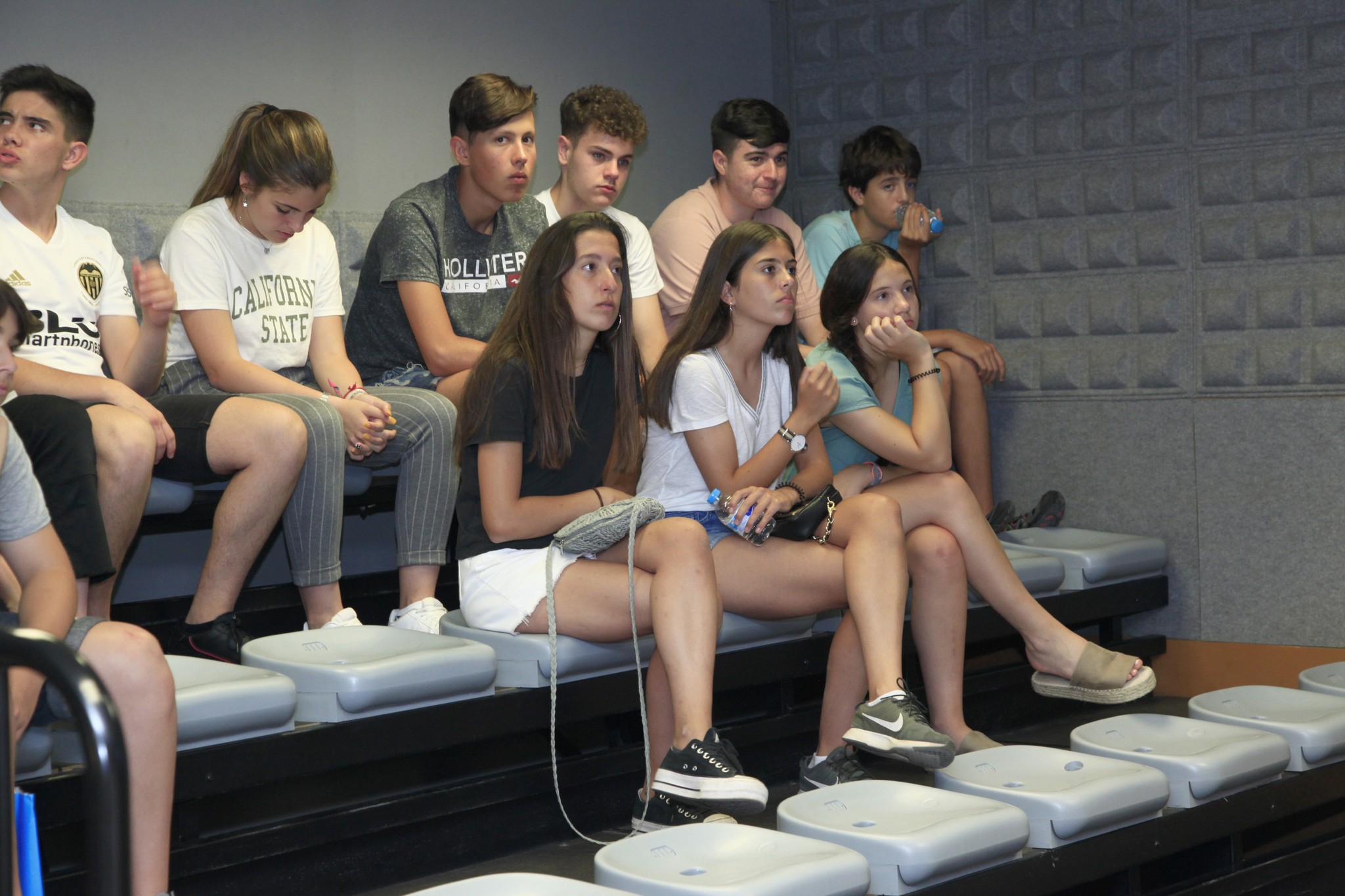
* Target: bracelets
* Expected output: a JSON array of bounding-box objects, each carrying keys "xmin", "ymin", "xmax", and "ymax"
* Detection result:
[
  {"xmin": 775, "ymin": 481, "xmax": 805, "ymax": 507},
  {"xmin": 328, "ymin": 377, "xmax": 369, "ymax": 400},
  {"xmin": 591, "ymin": 487, "xmax": 604, "ymax": 507},
  {"xmin": 320, "ymin": 391, "xmax": 331, "ymax": 402},
  {"xmin": 908, "ymin": 368, "xmax": 940, "ymax": 383}
]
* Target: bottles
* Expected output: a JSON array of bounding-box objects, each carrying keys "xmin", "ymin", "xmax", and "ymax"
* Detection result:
[
  {"xmin": 707, "ymin": 488, "xmax": 777, "ymax": 547},
  {"xmin": 895, "ymin": 203, "xmax": 944, "ymax": 233}
]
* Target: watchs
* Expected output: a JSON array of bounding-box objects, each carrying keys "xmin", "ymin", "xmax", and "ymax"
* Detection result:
[
  {"xmin": 777, "ymin": 425, "xmax": 808, "ymax": 453},
  {"xmin": 863, "ymin": 462, "xmax": 883, "ymax": 489}
]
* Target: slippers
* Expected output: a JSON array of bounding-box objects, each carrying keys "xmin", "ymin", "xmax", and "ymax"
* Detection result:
[
  {"xmin": 956, "ymin": 730, "xmax": 1005, "ymax": 757},
  {"xmin": 1031, "ymin": 640, "xmax": 1158, "ymax": 705}
]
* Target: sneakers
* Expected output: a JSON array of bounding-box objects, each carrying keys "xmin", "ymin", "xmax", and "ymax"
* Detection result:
[
  {"xmin": 985, "ymin": 499, "xmax": 1017, "ymax": 536},
  {"xmin": 175, "ymin": 613, "xmax": 254, "ymax": 664},
  {"xmin": 796, "ymin": 743, "xmax": 877, "ymax": 796},
  {"xmin": 302, "ymin": 607, "xmax": 363, "ymax": 632},
  {"xmin": 631, "ymin": 787, "xmax": 739, "ymax": 836},
  {"xmin": 841, "ymin": 676, "xmax": 956, "ymax": 768},
  {"xmin": 387, "ymin": 597, "xmax": 449, "ymax": 635},
  {"xmin": 650, "ymin": 728, "xmax": 770, "ymax": 818},
  {"xmin": 1018, "ymin": 491, "xmax": 1066, "ymax": 527}
]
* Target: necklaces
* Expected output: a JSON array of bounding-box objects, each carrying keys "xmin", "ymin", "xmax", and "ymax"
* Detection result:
[{"xmin": 235, "ymin": 207, "xmax": 273, "ymax": 254}]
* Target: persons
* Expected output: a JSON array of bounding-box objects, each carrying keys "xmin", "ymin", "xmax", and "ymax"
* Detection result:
[
  {"xmin": 0, "ymin": 63, "xmax": 307, "ymax": 667},
  {"xmin": 144, "ymin": 102, "xmax": 462, "ymax": 637},
  {"xmin": 532, "ymin": 83, "xmax": 667, "ymax": 396},
  {"xmin": 453, "ymin": 209, "xmax": 769, "ymax": 833},
  {"xmin": 343, "ymin": 70, "xmax": 551, "ymax": 405},
  {"xmin": 0, "ymin": 279, "xmax": 178, "ymax": 894},
  {"xmin": 633, "ymin": 218, "xmax": 956, "ymax": 789},
  {"xmin": 803, "ymin": 242, "xmax": 1157, "ymax": 754},
  {"xmin": 648, "ymin": 96, "xmax": 832, "ymax": 360},
  {"xmin": 802, "ymin": 127, "xmax": 1067, "ymax": 535}
]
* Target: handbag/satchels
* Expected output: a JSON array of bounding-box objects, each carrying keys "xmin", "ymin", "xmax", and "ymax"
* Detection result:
[
  {"xmin": 769, "ymin": 481, "xmax": 843, "ymax": 544},
  {"xmin": 14, "ymin": 780, "xmax": 45, "ymax": 896},
  {"xmin": 551, "ymin": 495, "xmax": 666, "ymax": 552}
]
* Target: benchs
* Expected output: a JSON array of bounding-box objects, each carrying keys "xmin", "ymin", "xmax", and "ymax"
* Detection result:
[{"xmin": 0, "ymin": 435, "xmax": 1345, "ymax": 896}]
